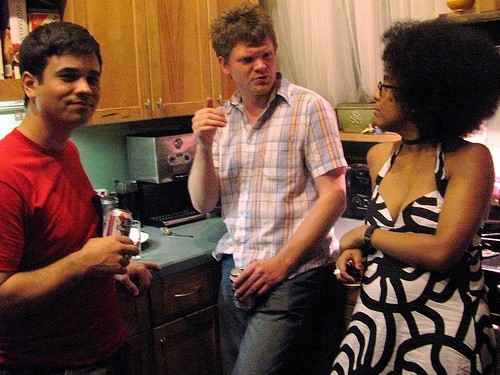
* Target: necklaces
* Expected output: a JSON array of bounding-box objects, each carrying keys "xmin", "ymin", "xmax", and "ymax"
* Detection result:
[{"xmin": 402, "ymin": 137, "xmax": 427, "ymax": 145}]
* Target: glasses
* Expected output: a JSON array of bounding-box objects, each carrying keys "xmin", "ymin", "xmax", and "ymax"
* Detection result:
[{"xmin": 377, "ymin": 81, "xmax": 400, "ymax": 93}]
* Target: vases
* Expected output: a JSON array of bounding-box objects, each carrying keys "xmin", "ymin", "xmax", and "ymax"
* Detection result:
[{"xmin": 334, "ymin": 102, "xmax": 377, "ymax": 134}]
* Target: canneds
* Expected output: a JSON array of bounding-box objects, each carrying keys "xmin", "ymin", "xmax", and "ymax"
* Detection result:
[{"xmin": 102, "ymin": 208, "xmax": 132, "ymax": 247}]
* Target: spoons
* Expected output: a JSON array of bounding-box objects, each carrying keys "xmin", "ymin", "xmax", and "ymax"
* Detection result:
[{"xmin": 160, "ymin": 227, "xmax": 194, "ymax": 238}]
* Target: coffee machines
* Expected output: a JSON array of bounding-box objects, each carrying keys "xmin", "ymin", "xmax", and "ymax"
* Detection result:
[{"xmin": 125, "ymin": 133, "xmax": 205, "ymax": 226}]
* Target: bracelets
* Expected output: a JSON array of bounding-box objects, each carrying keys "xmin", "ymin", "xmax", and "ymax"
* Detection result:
[{"xmin": 364, "ymin": 225, "xmax": 380, "ymax": 249}]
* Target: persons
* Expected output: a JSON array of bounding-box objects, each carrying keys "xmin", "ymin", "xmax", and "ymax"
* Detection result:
[
  {"xmin": 0, "ymin": 22, "xmax": 161, "ymax": 375},
  {"xmin": 188, "ymin": 7, "xmax": 351, "ymax": 375},
  {"xmin": 330, "ymin": 18, "xmax": 500, "ymax": 375}
]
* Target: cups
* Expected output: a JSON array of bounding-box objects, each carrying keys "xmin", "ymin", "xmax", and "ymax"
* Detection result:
[
  {"xmin": 125, "ymin": 220, "xmax": 141, "ymax": 260},
  {"xmin": 114, "ymin": 179, "xmax": 138, "ymax": 215}
]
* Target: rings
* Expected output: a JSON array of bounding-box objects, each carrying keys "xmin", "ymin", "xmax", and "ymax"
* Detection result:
[{"xmin": 333, "ymin": 269, "xmax": 341, "ymax": 275}]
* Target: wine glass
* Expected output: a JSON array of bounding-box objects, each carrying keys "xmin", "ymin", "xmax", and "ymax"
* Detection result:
[{"xmin": 348, "ymin": 163, "xmax": 372, "ymax": 209}]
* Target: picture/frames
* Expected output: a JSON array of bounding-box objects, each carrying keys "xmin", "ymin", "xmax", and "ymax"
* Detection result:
[{"xmin": 0, "ymin": 0, "xmax": 59, "ymax": 114}]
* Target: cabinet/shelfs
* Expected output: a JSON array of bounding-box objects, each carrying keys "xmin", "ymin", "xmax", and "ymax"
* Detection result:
[
  {"xmin": 116, "ymin": 214, "xmax": 500, "ymax": 375},
  {"xmin": 62, "ymin": 0, "xmax": 260, "ymax": 127}
]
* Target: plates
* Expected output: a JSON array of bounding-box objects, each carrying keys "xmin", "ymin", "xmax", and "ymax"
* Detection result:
[{"xmin": 129, "ymin": 227, "xmax": 149, "ymax": 245}]
